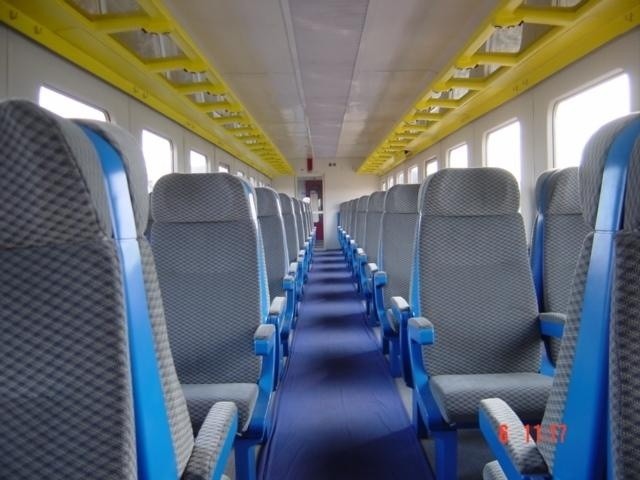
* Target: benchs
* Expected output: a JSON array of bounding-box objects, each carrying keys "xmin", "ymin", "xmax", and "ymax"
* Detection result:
[
  {"xmin": 337, "ymin": 112, "xmax": 640, "ymax": 480},
  {"xmin": 0, "ymin": 98, "xmax": 317, "ymax": 480}
]
